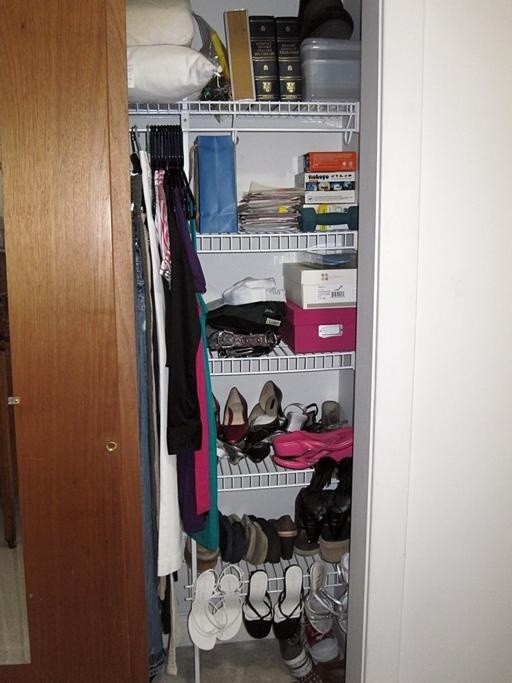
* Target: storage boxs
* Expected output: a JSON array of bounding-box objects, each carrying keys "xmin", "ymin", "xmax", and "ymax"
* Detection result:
[
  {"xmin": 275, "ymin": 260, "xmax": 360, "ymax": 356},
  {"xmin": 299, "ymin": 35, "xmax": 359, "ymax": 103}
]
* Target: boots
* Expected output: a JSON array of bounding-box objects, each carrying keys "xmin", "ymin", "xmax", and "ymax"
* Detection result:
[{"xmin": 298, "ymin": 0, "xmax": 354, "ymax": 45}]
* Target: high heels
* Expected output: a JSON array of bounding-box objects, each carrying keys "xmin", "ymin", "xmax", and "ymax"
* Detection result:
[
  {"xmin": 294, "ymin": 457, "xmax": 352, "ymax": 564},
  {"xmin": 246, "ymin": 381, "xmax": 284, "ymax": 462}
]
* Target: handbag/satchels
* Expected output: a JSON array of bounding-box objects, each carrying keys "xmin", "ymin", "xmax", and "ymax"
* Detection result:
[{"xmin": 188, "ymin": 134, "xmax": 238, "ymax": 234}]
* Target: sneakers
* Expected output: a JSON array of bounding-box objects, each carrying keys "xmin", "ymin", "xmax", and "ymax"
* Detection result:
[
  {"xmin": 184, "ymin": 514, "xmax": 298, "ymax": 572},
  {"xmin": 272, "ymin": 427, "xmax": 353, "ymax": 470},
  {"xmin": 279, "ymin": 619, "xmax": 340, "ymax": 680}
]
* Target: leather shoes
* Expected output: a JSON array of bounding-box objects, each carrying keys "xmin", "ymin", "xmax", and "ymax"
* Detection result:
[{"xmin": 211, "ymin": 384, "xmax": 249, "ymax": 465}]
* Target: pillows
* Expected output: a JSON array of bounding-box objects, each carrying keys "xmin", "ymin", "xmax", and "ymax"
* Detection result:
[{"xmin": 117, "ymin": 1, "xmax": 216, "ymax": 112}]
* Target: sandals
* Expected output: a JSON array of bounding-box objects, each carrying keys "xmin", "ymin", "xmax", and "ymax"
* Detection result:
[
  {"xmin": 273, "ymin": 563, "xmax": 304, "ymax": 641},
  {"xmin": 187, "ymin": 564, "xmax": 243, "ymax": 651},
  {"xmin": 304, "ymin": 561, "xmax": 334, "ymax": 635},
  {"xmin": 241, "ymin": 569, "xmax": 274, "ymax": 639},
  {"xmin": 336, "ymin": 551, "xmax": 350, "ymax": 635},
  {"xmin": 283, "ymin": 400, "xmax": 342, "ymax": 433}
]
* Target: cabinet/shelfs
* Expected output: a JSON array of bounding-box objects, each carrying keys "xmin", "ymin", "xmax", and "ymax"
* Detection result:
[{"xmin": 128, "ymin": 90, "xmax": 359, "ymax": 683}]
